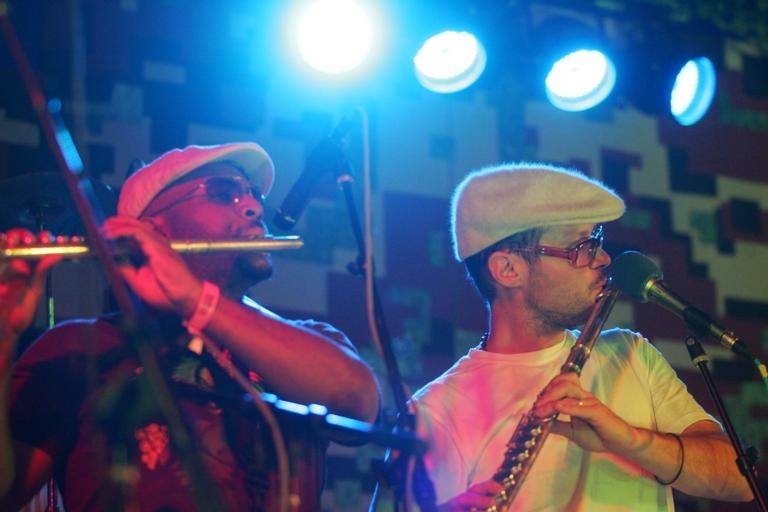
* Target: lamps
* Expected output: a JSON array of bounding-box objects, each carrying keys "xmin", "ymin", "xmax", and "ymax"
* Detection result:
[
  {"xmin": 405, "ymin": 16, "xmax": 488, "ymax": 96},
  {"xmin": 621, "ymin": 5, "xmax": 717, "ymax": 127},
  {"xmin": 524, "ymin": 0, "xmax": 620, "ymax": 113}
]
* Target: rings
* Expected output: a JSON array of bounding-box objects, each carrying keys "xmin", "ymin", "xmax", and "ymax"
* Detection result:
[{"xmin": 578, "ymin": 399, "xmax": 584, "ymax": 407}]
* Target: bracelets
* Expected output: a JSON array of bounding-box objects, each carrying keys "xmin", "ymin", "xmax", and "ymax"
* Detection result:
[
  {"xmin": 654, "ymin": 433, "xmax": 684, "ymax": 486},
  {"xmin": 181, "ymin": 280, "xmax": 220, "ymax": 336}
]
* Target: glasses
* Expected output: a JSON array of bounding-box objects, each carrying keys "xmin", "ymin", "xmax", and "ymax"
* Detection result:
[
  {"xmin": 493, "ymin": 224, "xmax": 606, "ymax": 270},
  {"xmin": 140, "ymin": 172, "xmax": 267, "ymax": 220}
]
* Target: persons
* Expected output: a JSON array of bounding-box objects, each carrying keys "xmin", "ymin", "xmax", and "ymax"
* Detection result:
[
  {"xmin": 0, "ymin": 142, "xmax": 383, "ymax": 512},
  {"xmin": 385, "ymin": 164, "xmax": 758, "ymax": 512}
]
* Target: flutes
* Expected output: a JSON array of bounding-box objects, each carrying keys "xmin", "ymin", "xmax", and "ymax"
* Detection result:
[
  {"xmin": 0, "ymin": 234, "xmax": 303, "ymax": 258},
  {"xmin": 478, "ymin": 282, "xmax": 618, "ymax": 511}
]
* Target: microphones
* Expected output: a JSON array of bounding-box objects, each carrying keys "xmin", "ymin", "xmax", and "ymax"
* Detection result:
[
  {"xmin": 611, "ymin": 251, "xmax": 754, "ymax": 362},
  {"xmin": 274, "ymin": 109, "xmax": 354, "ymax": 231}
]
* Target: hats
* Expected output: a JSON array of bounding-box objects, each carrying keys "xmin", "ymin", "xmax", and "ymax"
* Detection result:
[
  {"xmin": 448, "ymin": 161, "xmax": 627, "ymax": 264},
  {"xmin": 117, "ymin": 140, "xmax": 276, "ymax": 223}
]
